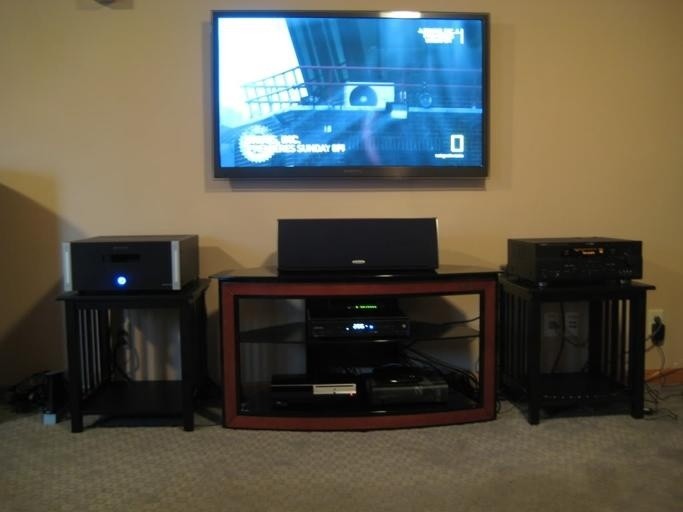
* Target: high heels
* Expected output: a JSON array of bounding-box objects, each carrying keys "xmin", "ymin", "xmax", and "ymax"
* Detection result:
[{"xmin": 7, "ymin": 369, "xmax": 48, "ymax": 409}]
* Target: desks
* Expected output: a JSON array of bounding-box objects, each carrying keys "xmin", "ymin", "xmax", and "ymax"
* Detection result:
[
  {"xmin": 500, "ymin": 275, "xmax": 656, "ymax": 426},
  {"xmin": 56, "ymin": 278, "xmax": 210, "ymax": 432}
]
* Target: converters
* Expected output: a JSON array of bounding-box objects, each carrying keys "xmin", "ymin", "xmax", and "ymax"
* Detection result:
[{"xmin": 651, "ymin": 324, "xmax": 665, "ymax": 345}]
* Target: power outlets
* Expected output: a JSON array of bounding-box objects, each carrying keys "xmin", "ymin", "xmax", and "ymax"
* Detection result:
[{"xmin": 646, "ymin": 309, "xmax": 662, "ymax": 347}]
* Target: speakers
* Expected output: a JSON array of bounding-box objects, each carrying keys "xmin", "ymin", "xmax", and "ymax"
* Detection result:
[{"xmin": 275, "ymin": 215, "xmax": 438, "ymax": 279}]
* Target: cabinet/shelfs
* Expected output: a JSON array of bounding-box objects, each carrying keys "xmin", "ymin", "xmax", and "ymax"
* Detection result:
[{"xmin": 218, "ymin": 266, "xmax": 504, "ymax": 431}]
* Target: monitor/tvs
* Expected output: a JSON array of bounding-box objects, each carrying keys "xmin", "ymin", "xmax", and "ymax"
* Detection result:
[{"xmin": 210, "ymin": 8, "xmax": 490, "ymax": 183}]
